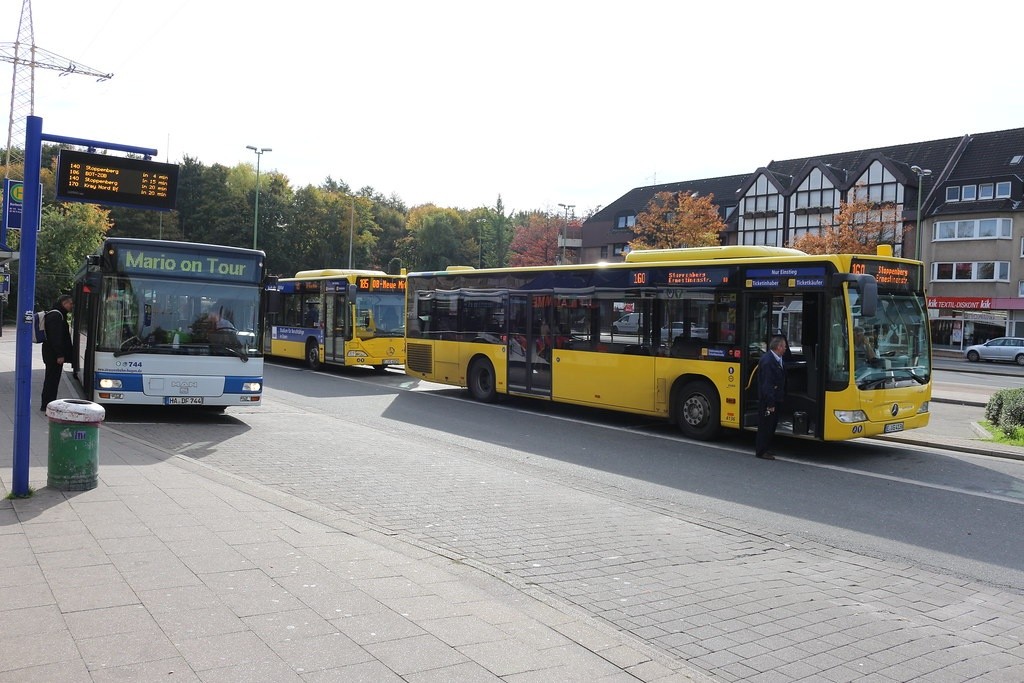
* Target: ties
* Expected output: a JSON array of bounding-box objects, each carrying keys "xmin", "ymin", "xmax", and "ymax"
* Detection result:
[{"xmin": 214, "ymin": 322, "xmax": 216, "ymax": 328}]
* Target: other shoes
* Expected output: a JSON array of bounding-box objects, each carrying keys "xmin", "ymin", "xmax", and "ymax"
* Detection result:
[{"xmin": 758, "ymin": 453, "xmax": 775, "ymax": 459}]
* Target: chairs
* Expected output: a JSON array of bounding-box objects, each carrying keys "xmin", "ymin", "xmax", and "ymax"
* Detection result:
[
  {"xmin": 771, "ymin": 328, "xmax": 808, "ymax": 390},
  {"xmin": 670, "ymin": 333, "xmax": 703, "ymax": 357}
]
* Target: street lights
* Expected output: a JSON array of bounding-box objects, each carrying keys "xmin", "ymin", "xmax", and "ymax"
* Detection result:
[
  {"xmin": 244, "ymin": 144, "xmax": 273, "ymax": 249},
  {"xmin": 558, "ymin": 203, "xmax": 577, "ymax": 266},
  {"xmin": 907, "ymin": 165, "xmax": 934, "ymax": 357},
  {"xmin": 476, "ymin": 218, "xmax": 487, "ymax": 269},
  {"xmin": 344, "ymin": 191, "xmax": 362, "ymax": 269}
]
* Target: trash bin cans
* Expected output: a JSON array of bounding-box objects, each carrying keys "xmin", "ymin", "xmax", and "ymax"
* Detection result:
[{"xmin": 46, "ymin": 398, "xmax": 105, "ymax": 490}]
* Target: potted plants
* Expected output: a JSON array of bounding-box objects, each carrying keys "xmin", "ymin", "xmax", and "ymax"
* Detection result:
[
  {"xmin": 743, "ymin": 210, "xmax": 777, "ymax": 218},
  {"xmin": 870, "ymin": 201, "xmax": 896, "ymax": 209},
  {"xmin": 794, "ymin": 206, "xmax": 831, "ymax": 215}
]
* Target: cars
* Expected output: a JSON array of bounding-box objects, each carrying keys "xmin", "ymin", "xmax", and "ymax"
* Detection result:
[
  {"xmin": 719, "ymin": 321, "xmax": 736, "ymax": 342},
  {"xmin": 963, "ymin": 335, "xmax": 1024, "ymax": 367},
  {"xmin": 649, "ymin": 320, "xmax": 709, "ymax": 343}
]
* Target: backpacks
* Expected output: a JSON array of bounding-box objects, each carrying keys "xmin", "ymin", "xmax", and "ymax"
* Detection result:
[{"xmin": 32, "ymin": 310, "xmax": 63, "ymax": 343}]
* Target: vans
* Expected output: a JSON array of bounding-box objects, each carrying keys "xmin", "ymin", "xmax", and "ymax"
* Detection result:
[{"xmin": 611, "ymin": 312, "xmax": 653, "ymax": 335}]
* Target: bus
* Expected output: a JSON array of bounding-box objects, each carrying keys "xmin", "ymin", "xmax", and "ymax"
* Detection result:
[
  {"xmin": 68, "ymin": 237, "xmax": 279, "ymax": 412},
  {"xmin": 264, "ymin": 266, "xmax": 408, "ymax": 372},
  {"xmin": 403, "ymin": 244, "xmax": 933, "ymax": 443}
]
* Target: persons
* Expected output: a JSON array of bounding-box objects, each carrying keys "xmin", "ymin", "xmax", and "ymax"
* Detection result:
[
  {"xmin": 756, "ymin": 337, "xmax": 786, "ymax": 460},
  {"xmin": 210, "ymin": 306, "xmax": 234, "ymax": 331},
  {"xmin": 40, "ymin": 295, "xmax": 74, "ymax": 411},
  {"xmin": 854, "ymin": 326, "xmax": 874, "ymax": 353},
  {"xmin": 305, "ymin": 303, "xmax": 319, "ymax": 328}
]
